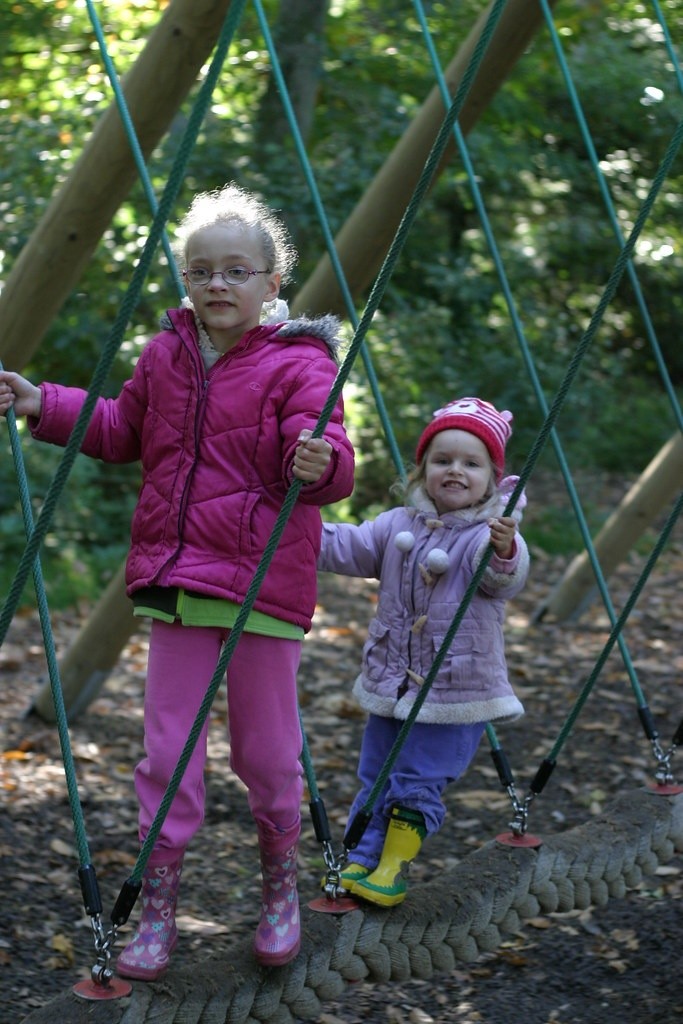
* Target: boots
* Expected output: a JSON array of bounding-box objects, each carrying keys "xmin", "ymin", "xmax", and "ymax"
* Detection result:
[
  {"xmin": 351, "ymin": 804, "xmax": 427, "ymax": 909},
  {"xmin": 321, "ymin": 861, "xmax": 374, "ymax": 892},
  {"xmin": 116, "ymin": 844, "xmax": 187, "ymax": 981},
  {"xmin": 252, "ymin": 813, "xmax": 299, "ymax": 964}
]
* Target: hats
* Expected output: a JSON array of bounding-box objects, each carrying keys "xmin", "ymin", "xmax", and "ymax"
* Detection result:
[{"xmin": 416, "ymin": 396, "xmax": 514, "ymax": 485}]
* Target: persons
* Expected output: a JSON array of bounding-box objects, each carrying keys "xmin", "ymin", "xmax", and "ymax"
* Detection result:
[
  {"xmin": 318, "ymin": 397, "xmax": 530, "ymax": 907},
  {"xmin": 0, "ymin": 180, "xmax": 355, "ymax": 981}
]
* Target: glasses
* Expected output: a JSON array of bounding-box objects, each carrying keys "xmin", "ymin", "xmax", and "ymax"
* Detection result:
[{"xmin": 182, "ymin": 265, "xmax": 270, "ymax": 286}]
